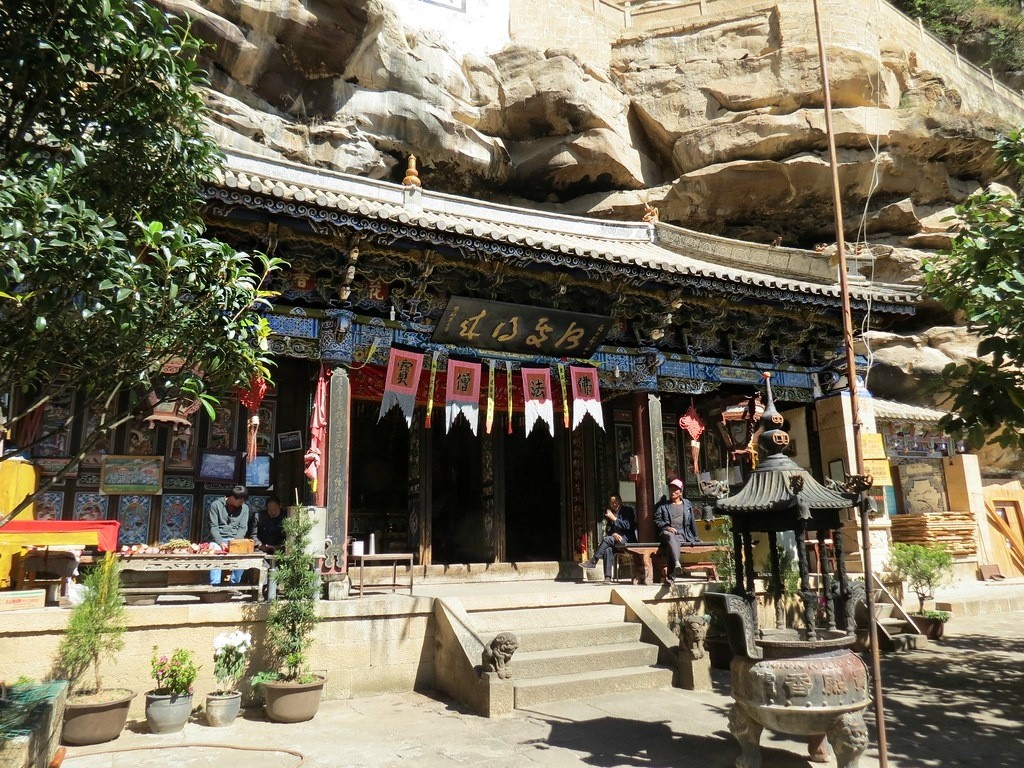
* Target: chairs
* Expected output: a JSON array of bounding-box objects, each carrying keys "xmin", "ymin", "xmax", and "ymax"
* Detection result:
[{"xmin": 805, "ymin": 528, "xmax": 837, "ymax": 575}]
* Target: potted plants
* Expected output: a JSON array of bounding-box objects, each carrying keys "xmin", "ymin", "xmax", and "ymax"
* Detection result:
[
  {"xmin": 168, "ymin": 538, "xmax": 192, "ymax": 554},
  {"xmin": 248, "ymin": 485, "xmax": 328, "ymax": 724},
  {"xmin": 51, "ymin": 550, "xmax": 139, "ymax": 745},
  {"xmin": 888, "ymin": 540, "xmax": 955, "ymax": 641}
]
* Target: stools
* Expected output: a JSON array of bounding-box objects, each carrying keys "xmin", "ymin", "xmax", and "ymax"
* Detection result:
[{"xmin": 613, "ymin": 544, "xmax": 637, "ymax": 585}]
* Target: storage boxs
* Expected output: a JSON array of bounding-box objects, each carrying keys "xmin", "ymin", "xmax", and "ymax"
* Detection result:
[
  {"xmin": 0, "ymin": 588, "xmax": 47, "ymax": 612},
  {"xmin": 228, "ymin": 539, "xmax": 255, "ymax": 553}
]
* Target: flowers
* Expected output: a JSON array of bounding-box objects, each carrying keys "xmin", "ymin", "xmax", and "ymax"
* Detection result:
[
  {"xmin": 212, "ymin": 631, "xmax": 253, "ymax": 697},
  {"xmin": 150, "ymin": 644, "xmax": 204, "ymax": 699}
]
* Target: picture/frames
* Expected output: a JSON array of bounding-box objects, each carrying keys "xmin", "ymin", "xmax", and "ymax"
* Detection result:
[
  {"xmin": 98, "ymin": 454, "xmax": 165, "ymax": 496},
  {"xmin": 193, "ymin": 448, "xmax": 242, "ymax": 485},
  {"xmin": 277, "ymin": 431, "xmax": 303, "ymax": 455},
  {"xmin": 241, "ymin": 452, "xmax": 275, "ymax": 491}
]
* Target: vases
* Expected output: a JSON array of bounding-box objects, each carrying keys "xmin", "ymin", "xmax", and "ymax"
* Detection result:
[
  {"xmin": 145, "ymin": 688, "xmax": 195, "ymax": 734},
  {"xmin": 205, "ymin": 690, "xmax": 242, "ymax": 727}
]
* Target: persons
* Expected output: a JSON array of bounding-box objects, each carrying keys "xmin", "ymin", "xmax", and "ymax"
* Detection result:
[
  {"xmin": 577, "ymin": 492, "xmax": 640, "ymax": 584},
  {"xmin": 651, "ymin": 479, "xmax": 705, "ymax": 587},
  {"xmin": 207, "ymin": 485, "xmax": 250, "ymax": 587},
  {"xmin": 246, "ymin": 495, "xmax": 288, "ymax": 565}
]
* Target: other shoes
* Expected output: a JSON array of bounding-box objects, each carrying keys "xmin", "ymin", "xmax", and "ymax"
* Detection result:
[
  {"xmin": 667, "ymin": 575, "xmax": 674, "ymax": 585},
  {"xmin": 674, "ymin": 565, "xmax": 682, "ymax": 576},
  {"xmin": 603, "ymin": 577, "xmax": 611, "ymax": 584},
  {"xmin": 578, "ymin": 562, "xmax": 596, "ymax": 569}
]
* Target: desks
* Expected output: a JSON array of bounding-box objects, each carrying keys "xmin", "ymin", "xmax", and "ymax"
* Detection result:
[
  {"xmin": 1, "ymin": 521, "xmax": 121, "ymax": 608},
  {"xmin": 117, "ymin": 552, "xmax": 270, "ymax": 603},
  {"xmin": 348, "ymin": 554, "xmax": 414, "ymax": 598}
]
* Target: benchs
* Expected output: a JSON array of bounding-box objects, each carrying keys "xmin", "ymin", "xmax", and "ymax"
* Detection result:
[{"xmin": 617, "ymin": 540, "xmax": 730, "ymax": 586}]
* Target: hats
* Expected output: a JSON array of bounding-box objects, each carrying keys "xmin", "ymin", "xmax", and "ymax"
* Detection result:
[{"xmin": 668, "ymin": 479, "xmax": 684, "ymax": 489}]
